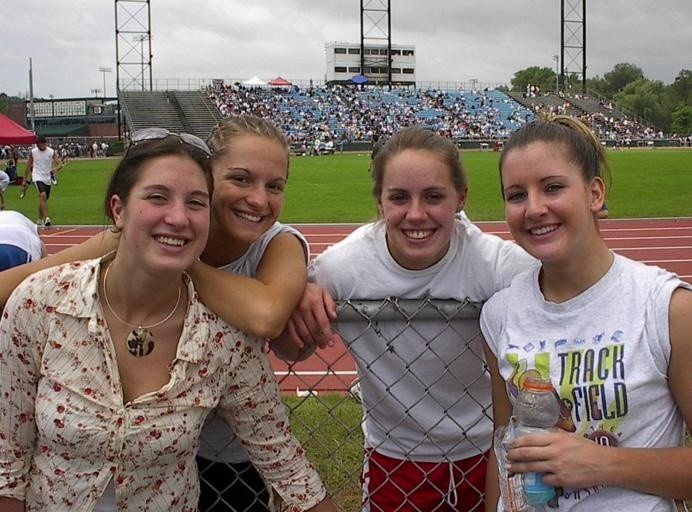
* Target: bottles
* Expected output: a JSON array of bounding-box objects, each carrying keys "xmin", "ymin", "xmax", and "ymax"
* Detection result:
[{"xmin": 513, "ymin": 374, "xmax": 560, "ymax": 504}]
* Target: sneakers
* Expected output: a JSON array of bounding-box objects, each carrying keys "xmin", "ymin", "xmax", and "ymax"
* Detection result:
[{"xmin": 43, "ymin": 215, "xmax": 54, "ymax": 229}]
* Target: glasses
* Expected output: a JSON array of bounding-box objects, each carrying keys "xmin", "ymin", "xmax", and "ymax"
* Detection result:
[{"xmin": 127, "ymin": 119, "xmax": 223, "ymax": 160}]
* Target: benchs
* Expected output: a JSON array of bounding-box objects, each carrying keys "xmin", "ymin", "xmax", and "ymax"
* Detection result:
[{"xmin": 121, "ymin": 90, "xmax": 662, "ymax": 143}]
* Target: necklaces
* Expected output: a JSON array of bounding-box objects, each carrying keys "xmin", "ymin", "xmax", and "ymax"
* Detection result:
[{"xmin": 102, "ymin": 256, "xmax": 183, "ymax": 360}]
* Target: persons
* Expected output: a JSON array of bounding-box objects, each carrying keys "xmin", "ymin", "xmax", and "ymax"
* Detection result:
[
  {"xmin": 0, "ymin": 143, "xmax": 33, "ymax": 210},
  {"xmin": 0, "ymin": 208, "xmax": 50, "ymax": 274},
  {"xmin": 201, "ymin": 83, "xmax": 692, "ymax": 159},
  {"xmin": 0, "ymin": 135, "xmax": 111, "ymax": 161},
  {"xmin": 262, "ymin": 123, "xmax": 538, "ymax": 511},
  {"xmin": 475, "ymin": 114, "xmax": 690, "ymax": 511},
  {"xmin": 594, "ymin": 202, "xmax": 610, "ymax": 220},
  {"xmin": 22, "ymin": 135, "xmax": 65, "ymax": 228}
]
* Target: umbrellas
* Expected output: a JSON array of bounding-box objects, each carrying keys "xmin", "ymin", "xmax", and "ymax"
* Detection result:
[
  {"xmin": 242, "ymin": 76, "xmax": 266, "ymax": 88},
  {"xmin": 351, "ymin": 75, "xmax": 369, "ymax": 86},
  {"xmin": 267, "ymin": 76, "xmax": 292, "ymax": 88}
]
